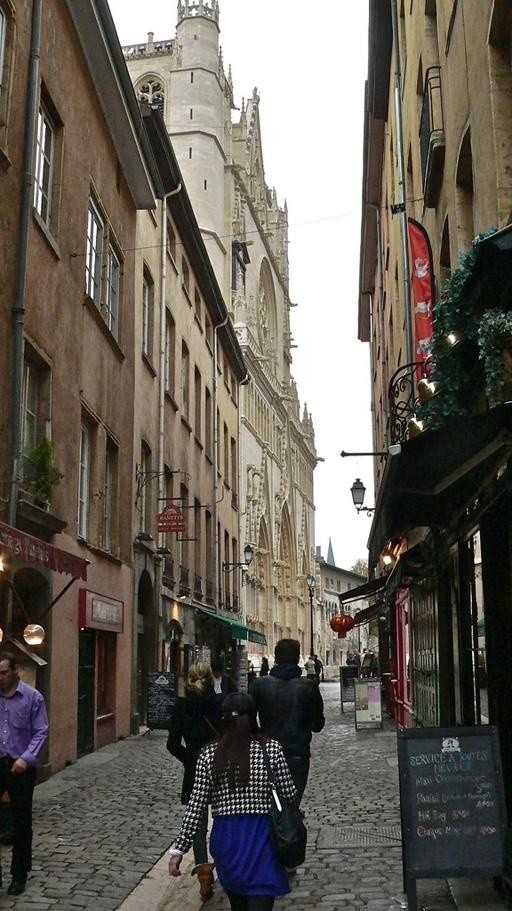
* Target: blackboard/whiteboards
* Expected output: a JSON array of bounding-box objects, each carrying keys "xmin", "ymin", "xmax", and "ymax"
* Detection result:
[
  {"xmin": 146, "ymin": 670, "xmax": 177, "ymax": 728},
  {"xmin": 340, "ymin": 666, "xmax": 360, "ymax": 702},
  {"xmin": 397, "ymin": 724, "xmax": 511, "ymax": 878}
]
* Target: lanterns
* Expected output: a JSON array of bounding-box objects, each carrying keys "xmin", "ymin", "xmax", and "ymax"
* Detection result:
[{"xmin": 330, "ymin": 611, "xmax": 354, "ymax": 638}]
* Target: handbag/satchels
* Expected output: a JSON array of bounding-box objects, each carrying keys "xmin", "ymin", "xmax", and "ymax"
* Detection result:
[{"xmin": 272, "ymin": 807, "xmax": 307, "ymax": 868}]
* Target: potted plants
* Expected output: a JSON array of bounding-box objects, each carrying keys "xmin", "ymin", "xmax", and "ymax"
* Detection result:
[{"xmin": 13, "ymin": 436, "xmax": 66, "ymax": 511}]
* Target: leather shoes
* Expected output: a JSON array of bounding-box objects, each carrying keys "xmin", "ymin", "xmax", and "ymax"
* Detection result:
[{"xmin": 7, "ymin": 872, "xmax": 27, "ymax": 895}]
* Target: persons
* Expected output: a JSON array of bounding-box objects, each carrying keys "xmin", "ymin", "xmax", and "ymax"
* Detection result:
[
  {"xmin": 346, "ymin": 648, "xmax": 375, "ymax": 678},
  {"xmin": 0, "ymin": 651, "xmax": 50, "ymax": 895},
  {"xmin": 166, "ymin": 641, "xmax": 326, "ymax": 911}
]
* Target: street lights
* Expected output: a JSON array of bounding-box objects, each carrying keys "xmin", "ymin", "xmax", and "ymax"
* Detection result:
[
  {"xmin": 354, "ymin": 608, "xmax": 362, "ymax": 653},
  {"xmin": 306, "ymin": 573, "xmax": 315, "ymax": 661}
]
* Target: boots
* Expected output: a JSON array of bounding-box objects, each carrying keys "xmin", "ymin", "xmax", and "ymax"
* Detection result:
[{"xmin": 192, "ymin": 863, "xmax": 216, "ymax": 900}]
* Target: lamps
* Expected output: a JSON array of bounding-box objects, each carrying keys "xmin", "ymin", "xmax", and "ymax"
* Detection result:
[
  {"xmin": 382, "ymin": 553, "xmax": 393, "ymax": 575},
  {"xmin": 222, "ymin": 545, "xmax": 255, "ymax": 573},
  {"xmin": 406, "ymin": 327, "xmax": 462, "ymax": 440},
  {"xmin": 156, "ymin": 547, "xmax": 172, "ymax": 554},
  {"xmin": 350, "ymin": 478, "xmax": 376, "ymax": 517},
  {"xmin": 134, "ymin": 533, "xmax": 153, "ymax": 542}
]
